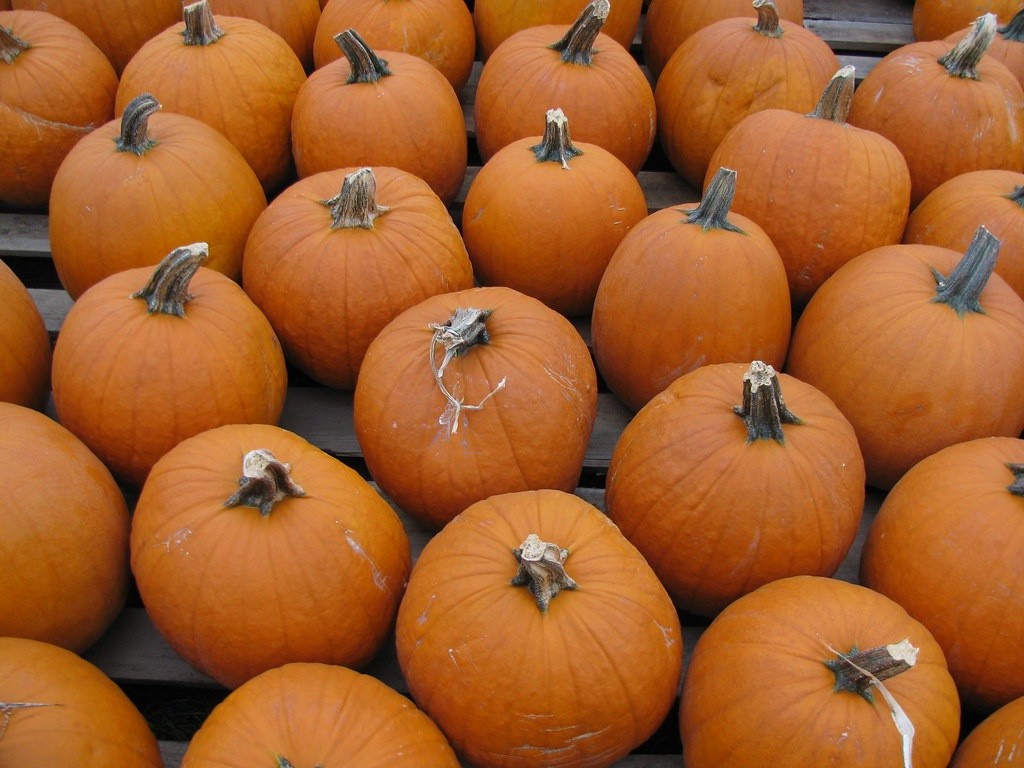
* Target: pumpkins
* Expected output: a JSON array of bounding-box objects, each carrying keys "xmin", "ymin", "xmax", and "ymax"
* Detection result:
[{"xmin": 0, "ymin": 0, "xmax": 1024, "ymax": 768}]
